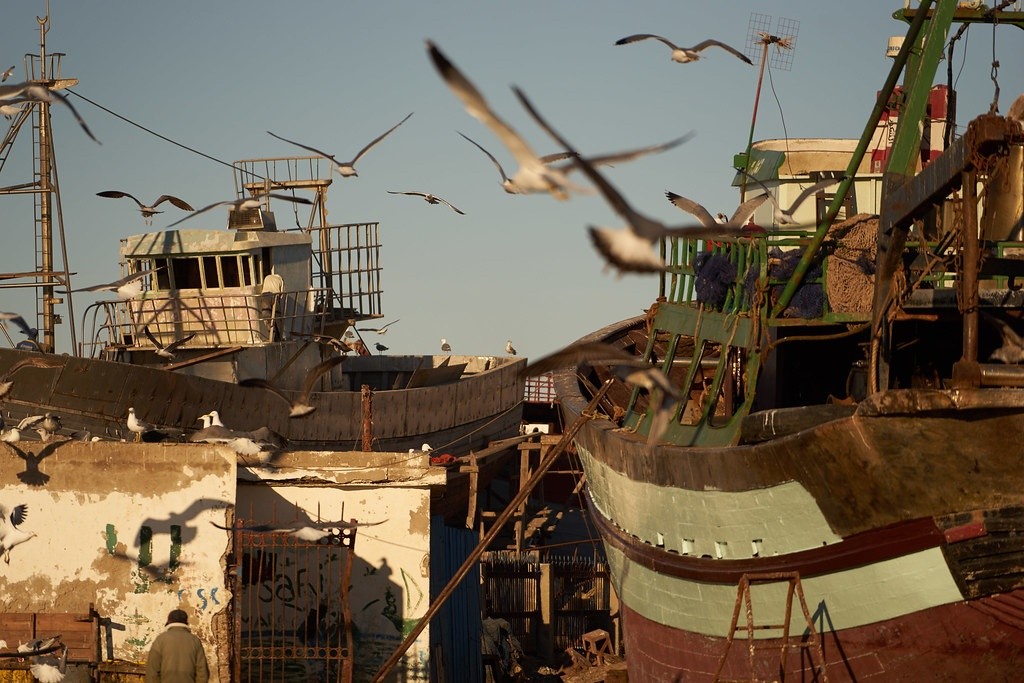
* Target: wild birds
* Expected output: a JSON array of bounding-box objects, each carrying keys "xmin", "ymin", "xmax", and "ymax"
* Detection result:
[{"xmin": 0, "ymin": 30, "xmax": 799, "ymax": 683}]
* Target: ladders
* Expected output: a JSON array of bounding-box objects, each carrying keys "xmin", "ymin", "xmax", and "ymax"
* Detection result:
[{"xmin": 373, "ymin": 376, "xmax": 614, "ymax": 683}]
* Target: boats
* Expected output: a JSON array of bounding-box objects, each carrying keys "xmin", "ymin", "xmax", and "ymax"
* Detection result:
[
  {"xmin": 0, "ymin": 16, "xmax": 529, "ymax": 466},
  {"xmin": 731, "ymin": 138, "xmax": 986, "ymax": 241},
  {"xmin": 552, "ymin": 0, "xmax": 1024, "ymax": 683}
]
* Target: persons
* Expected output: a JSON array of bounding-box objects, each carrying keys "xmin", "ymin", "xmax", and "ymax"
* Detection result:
[
  {"xmin": 17, "ymin": 328, "xmax": 39, "ymax": 351},
  {"xmin": 531, "ymin": 428, "xmax": 541, "ymax": 443},
  {"xmin": 262, "ymin": 274, "xmax": 283, "ymax": 339},
  {"xmin": 144, "ymin": 610, "xmax": 209, "ymax": 683}
]
